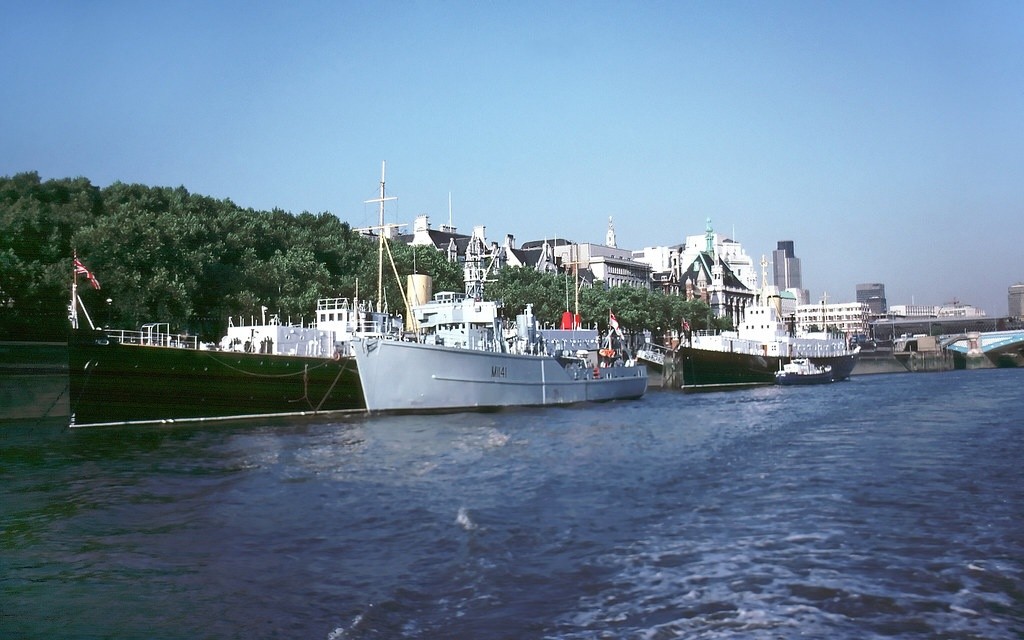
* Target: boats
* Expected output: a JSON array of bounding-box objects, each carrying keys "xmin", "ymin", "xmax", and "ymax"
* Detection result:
[
  {"xmin": 667, "ymin": 255, "xmax": 862, "ymax": 394},
  {"xmin": 774, "ymin": 351, "xmax": 833, "ymax": 386},
  {"xmin": 63, "ymin": 160, "xmax": 653, "ymax": 431}
]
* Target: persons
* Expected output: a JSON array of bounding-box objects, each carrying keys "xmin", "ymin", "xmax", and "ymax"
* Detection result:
[{"xmin": 593, "ymin": 364, "xmax": 600, "ymax": 378}]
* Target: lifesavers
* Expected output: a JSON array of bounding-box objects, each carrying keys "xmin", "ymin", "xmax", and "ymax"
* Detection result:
[{"xmin": 333, "ymin": 352, "xmax": 340, "ymax": 361}]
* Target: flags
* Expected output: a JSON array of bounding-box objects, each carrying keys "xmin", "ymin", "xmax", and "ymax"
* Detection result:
[
  {"xmin": 610, "ymin": 313, "xmax": 624, "ymax": 340},
  {"xmin": 75, "ymin": 258, "xmax": 100, "ymax": 290},
  {"xmin": 681, "ymin": 319, "xmax": 690, "ymax": 331}
]
